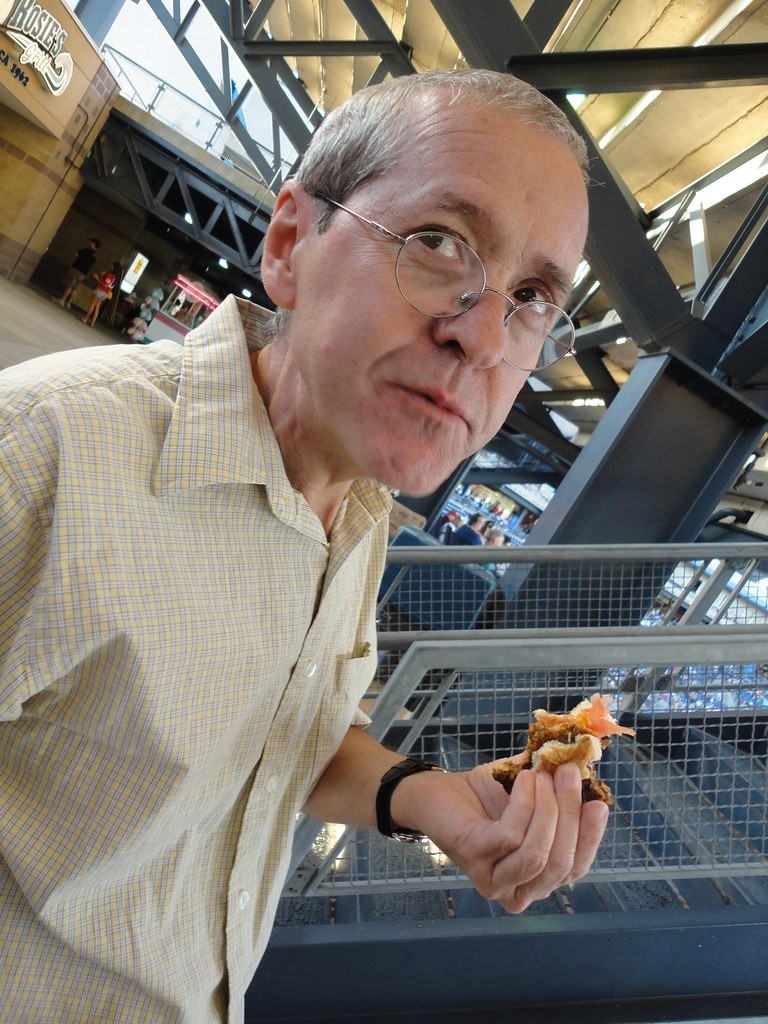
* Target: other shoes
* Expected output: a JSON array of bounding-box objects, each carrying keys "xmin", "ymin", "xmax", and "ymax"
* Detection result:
[
  {"xmin": 65, "ymin": 302, "xmax": 71, "ymax": 310},
  {"xmin": 76, "ymin": 316, "xmax": 87, "ymax": 323},
  {"xmin": 52, "ymin": 296, "xmax": 65, "ymax": 307},
  {"xmin": 89, "ymin": 320, "xmax": 94, "ymax": 327}
]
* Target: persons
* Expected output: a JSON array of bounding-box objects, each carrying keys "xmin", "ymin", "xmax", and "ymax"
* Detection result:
[
  {"xmin": 51, "ymin": 236, "xmax": 120, "ymax": 328},
  {"xmin": 0, "ymin": 66, "xmax": 610, "ymax": 1024},
  {"xmin": 428, "ymin": 509, "xmax": 514, "ymax": 576}
]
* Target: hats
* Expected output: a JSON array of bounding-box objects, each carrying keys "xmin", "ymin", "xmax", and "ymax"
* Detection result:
[
  {"xmin": 447, "ymin": 510, "xmax": 457, "ymax": 517},
  {"xmin": 90, "ymin": 237, "xmax": 100, "ymax": 248}
]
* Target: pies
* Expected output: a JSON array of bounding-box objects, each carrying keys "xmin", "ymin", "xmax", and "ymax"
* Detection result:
[{"xmin": 491, "ymin": 694, "xmax": 631, "ymax": 805}]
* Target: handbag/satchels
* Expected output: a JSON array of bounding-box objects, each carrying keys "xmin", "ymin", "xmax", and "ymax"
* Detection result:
[{"xmin": 106, "ymin": 290, "xmax": 113, "ymax": 300}]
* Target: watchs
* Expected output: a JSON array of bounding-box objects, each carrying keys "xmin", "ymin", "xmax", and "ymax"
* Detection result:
[{"xmin": 376, "ymin": 758, "xmax": 449, "ymax": 844}]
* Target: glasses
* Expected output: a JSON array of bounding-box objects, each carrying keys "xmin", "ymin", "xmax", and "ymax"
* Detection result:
[{"xmin": 307, "ymin": 188, "xmax": 577, "ymax": 372}]
